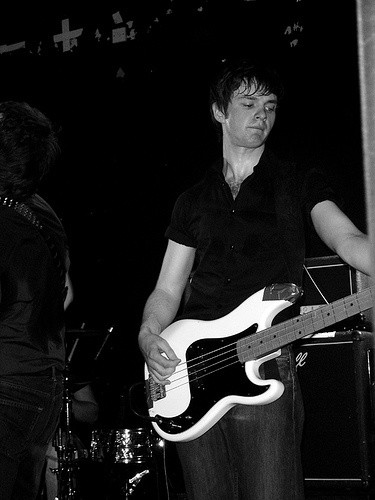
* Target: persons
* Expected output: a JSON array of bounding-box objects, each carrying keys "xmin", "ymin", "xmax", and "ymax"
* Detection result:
[
  {"xmin": 0, "ymin": 102, "xmax": 68, "ymax": 500},
  {"xmin": 138, "ymin": 67, "xmax": 375, "ymax": 500}
]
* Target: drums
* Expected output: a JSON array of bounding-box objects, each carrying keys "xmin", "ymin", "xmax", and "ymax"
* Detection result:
[{"xmin": 115, "ymin": 429, "xmax": 153, "ymax": 464}]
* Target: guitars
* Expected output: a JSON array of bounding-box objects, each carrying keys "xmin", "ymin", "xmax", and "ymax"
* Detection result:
[
  {"xmin": 78, "ymin": 428, "xmax": 110, "ymax": 466},
  {"xmin": 144, "ymin": 267, "xmax": 371, "ymax": 441}
]
